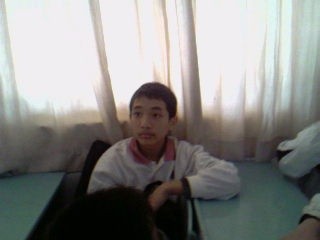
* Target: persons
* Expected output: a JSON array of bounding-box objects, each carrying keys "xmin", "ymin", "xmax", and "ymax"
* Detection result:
[
  {"xmin": 277, "ymin": 193, "xmax": 320, "ymax": 240},
  {"xmin": 46, "ymin": 184, "xmax": 157, "ymax": 240},
  {"xmin": 88, "ymin": 82, "xmax": 241, "ymax": 214}
]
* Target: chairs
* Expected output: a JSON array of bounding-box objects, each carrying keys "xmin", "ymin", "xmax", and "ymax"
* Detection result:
[{"xmin": 71, "ymin": 139, "xmax": 188, "ymax": 240}]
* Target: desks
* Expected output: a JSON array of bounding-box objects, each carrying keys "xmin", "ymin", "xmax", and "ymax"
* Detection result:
[
  {"xmin": 0, "ymin": 170, "xmax": 67, "ymax": 239},
  {"xmin": 188, "ymin": 162, "xmax": 320, "ymax": 240}
]
